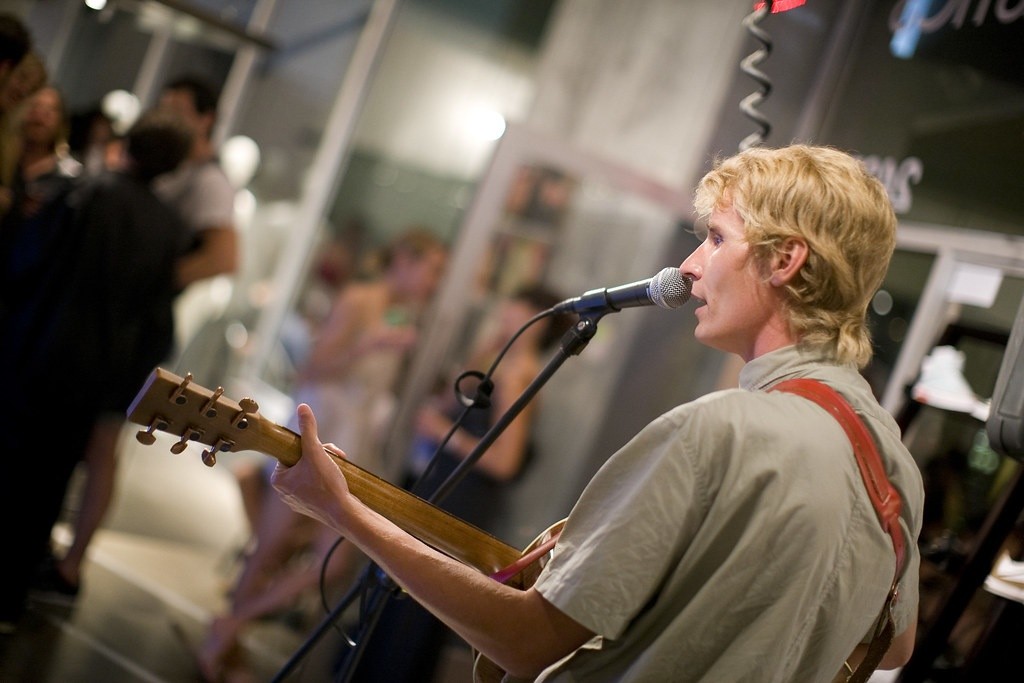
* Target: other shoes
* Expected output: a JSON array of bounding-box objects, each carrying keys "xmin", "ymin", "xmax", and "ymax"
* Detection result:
[{"xmin": 34, "ymin": 566, "xmax": 79, "ymax": 596}]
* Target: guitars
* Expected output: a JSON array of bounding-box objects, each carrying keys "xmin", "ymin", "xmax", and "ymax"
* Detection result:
[{"xmin": 125, "ymin": 364, "xmax": 568, "ymax": 683}]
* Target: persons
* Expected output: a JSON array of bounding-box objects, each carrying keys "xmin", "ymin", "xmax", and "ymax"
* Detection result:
[
  {"xmin": 0, "ymin": 9, "xmax": 194, "ymax": 620},
  {"xmin": 328, "ymin": 285, "xmax": 572, "ymax": 682},
  {"xmin": 271, "ymin": 143, "xmax": 925, "ymax": 682},
  {"xmin": 58, "ymin": 73, "xmax": 236, "ymax": 594},
  {"xmin": 170, "ymin": 229, "xmax": 451, "ymax": 682}
]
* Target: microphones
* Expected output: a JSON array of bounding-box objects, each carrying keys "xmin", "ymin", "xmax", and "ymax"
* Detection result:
[{"xmin": 552, "ymin": 267, "xmax": 694, "ymax": 315}]
